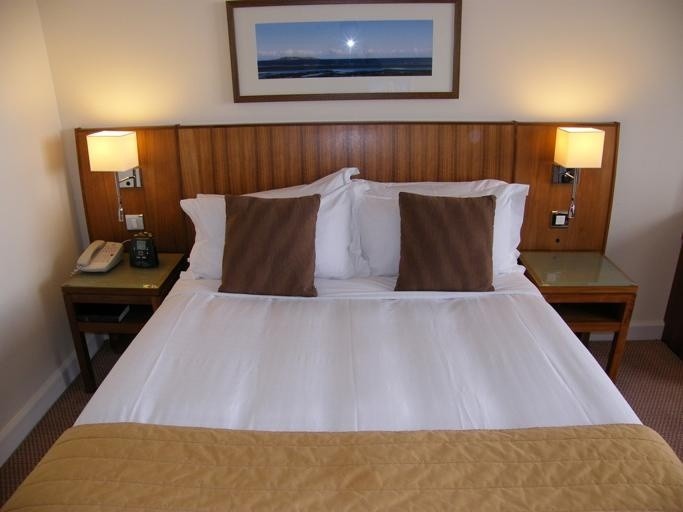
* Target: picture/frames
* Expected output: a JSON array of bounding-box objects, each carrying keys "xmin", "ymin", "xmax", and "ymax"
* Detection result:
[{"xmin": 226, "ymin": 0, "xmax": 461, "ymax": 104}]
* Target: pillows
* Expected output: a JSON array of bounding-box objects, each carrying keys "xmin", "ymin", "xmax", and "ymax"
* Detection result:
[
  {"xmin": 352, "ymin": 177, "xmax": 530, "ymax": 290},
  {"xmin": 179, "ymin": 167, "xmax": 370, "ymax": 296}
]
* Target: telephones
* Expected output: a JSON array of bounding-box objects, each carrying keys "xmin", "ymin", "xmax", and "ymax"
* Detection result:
[{"xmin": 75, "ymin": 240, "xmax": 124, "ymax": 272}]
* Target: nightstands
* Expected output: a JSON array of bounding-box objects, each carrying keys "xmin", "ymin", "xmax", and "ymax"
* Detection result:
[
  {"xmin": 517, "ymin": 251, "xmax": 640, "ymax": 385},
  {"xmin": 59, "ymin": 251, "xmax": 186, "ymax": 394}
]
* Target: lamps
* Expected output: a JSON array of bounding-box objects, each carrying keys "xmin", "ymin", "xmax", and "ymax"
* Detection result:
[
  {"xmin": 551, "ymin": 126, "xmax": 606, "ymax": 219},
  {"xmin": 85, "ymin": 129, "xmax": 144, "ymax": 223}
]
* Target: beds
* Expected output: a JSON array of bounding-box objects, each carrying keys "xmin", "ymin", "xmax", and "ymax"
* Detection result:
[{"xmin": 0, "ymin": 120, "xmax": 683, "ymax": 511}]
